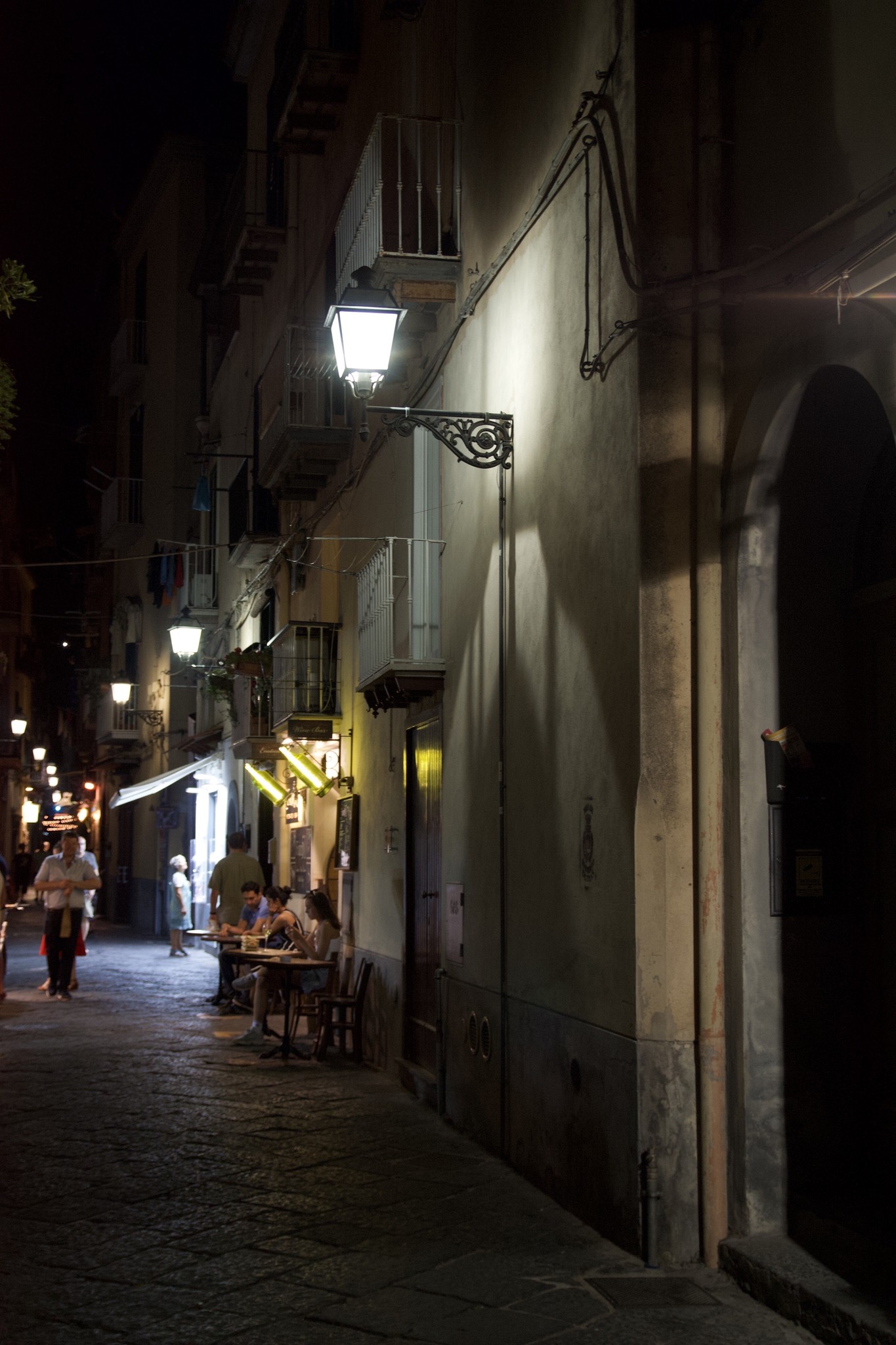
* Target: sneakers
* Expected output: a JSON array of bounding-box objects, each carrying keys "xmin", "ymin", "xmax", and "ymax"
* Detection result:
[
  {"xmin": 234, "ymin": 1029, "xmax": 264, "ymax": 1045},
  {"xmin": 232, "ymin": 973, "xmax": 256, "ymax": 991}
]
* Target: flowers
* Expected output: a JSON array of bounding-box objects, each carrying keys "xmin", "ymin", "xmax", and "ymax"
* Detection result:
[{"xmin": 224, "ymin": 647, "xmax": 262, "ymax": 688}]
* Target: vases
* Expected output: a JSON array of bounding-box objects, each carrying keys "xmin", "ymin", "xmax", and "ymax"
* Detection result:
[{"xmin": 231, "ymin": 663, "xmax": 266, "ymax": 677}]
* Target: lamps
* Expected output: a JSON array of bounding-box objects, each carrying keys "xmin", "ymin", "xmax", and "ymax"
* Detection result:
[
  {"xmin": 244, "ymin": 757, "xmax": 294, "ymax": 808},
  {"xmin": 279, "ymin": 737, "xmax": 342, "ymax": 798},
  {"xmin": 167, "ymin": 605, "xmax": 228, "ymax": 674},
  {"xmin": 323, "ymin": 265, "xmax": 514, "ymax": 469},
  {"xmin": 109, "ymin": 668, "xmax": 163, "ymax": 726},
  {"xmin": 0, "ymin": 705, "xmax": 46, "ymax": 772}
]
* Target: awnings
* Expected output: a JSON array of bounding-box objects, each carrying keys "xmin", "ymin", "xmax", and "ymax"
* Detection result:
[{"xmin": 109, "ymin": 755, "xmax": 215, "ymax": 809}]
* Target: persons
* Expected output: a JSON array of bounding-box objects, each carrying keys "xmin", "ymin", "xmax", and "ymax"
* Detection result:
[
  {"xmin": 204, "ymin": 880, "xmax": 305, "ymax": 1016},
  {"xmin": 208, "ymin": 832, "xmax": 267, "ymax": 1004},
  {"xmin": 34, "ymin": 831, "xmax": 101, "ymax": 1001},
  {"xmin": 230, "ymin": 887, "xmax": 343, "ymax": 1046},
  {"xmin": 166, "ymin": 854, "xmax": 193, "ymax": 958},
  {"xmin": 0, "ymin": 869, "xmax": 8, "ymax": 1002},
  {"xmin": 36, "ymin": 835, "xmax": 99, "ymax": 992}
]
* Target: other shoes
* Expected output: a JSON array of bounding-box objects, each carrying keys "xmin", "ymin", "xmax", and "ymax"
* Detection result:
[
  {"xmin": 68, "ymin": 981, "xmax": 78, "ymax": 991},
  {"xmin": 177, "ymin": 950, "xmax": 188, "ymax": 956},
  {"xmin": 170, "ymin": 950, "xmax": 184, "ymax": 957},
  {"xmin": 46, "ymin": 979, "xmax": 57, "ymax": 996},
  {"xmin": 37, "ymin": 979, "xmax": 60, "ymax": 991},
  {"xmin": 56, "ymin": 987, "xmax": 72, "ymax": 1000}
]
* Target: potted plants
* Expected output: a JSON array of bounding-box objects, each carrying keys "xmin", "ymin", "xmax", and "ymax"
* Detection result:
[{"xmin": 201, "ymin": 668, "xmax": 240, "ymax": 728}]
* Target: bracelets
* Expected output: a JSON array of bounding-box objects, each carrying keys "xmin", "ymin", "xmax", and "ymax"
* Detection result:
[{"xmin": 210, "ymin": 912, "xmax": 216, "ymax": 915}]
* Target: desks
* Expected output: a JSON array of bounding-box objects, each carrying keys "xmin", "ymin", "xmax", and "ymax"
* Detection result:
[
  {"xmin": 244, "ymin": 957, "xmax": 334, "ymax": 1060},
  {"xmin": 201, "ymin": 934, "xmax": 266, "ymax": 1002},
  {"xmin": 227, "ymin": 948, "xmax": 302, "ymax": 1039}
]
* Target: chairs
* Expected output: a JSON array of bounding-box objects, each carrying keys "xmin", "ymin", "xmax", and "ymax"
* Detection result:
[{"xmin": 290, "ymin": 950, "xmax": 374, "ymax": 1060}]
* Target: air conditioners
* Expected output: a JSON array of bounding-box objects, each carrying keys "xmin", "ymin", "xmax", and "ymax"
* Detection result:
[{"xmin": 190, "ymin": 574, "xmax": 213, "ymax": 607}]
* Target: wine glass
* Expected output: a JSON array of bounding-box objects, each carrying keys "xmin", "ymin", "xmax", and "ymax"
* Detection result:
[{"xmin": 262, "ymin": 923, "xmax": 272, "ymax": 952}]
